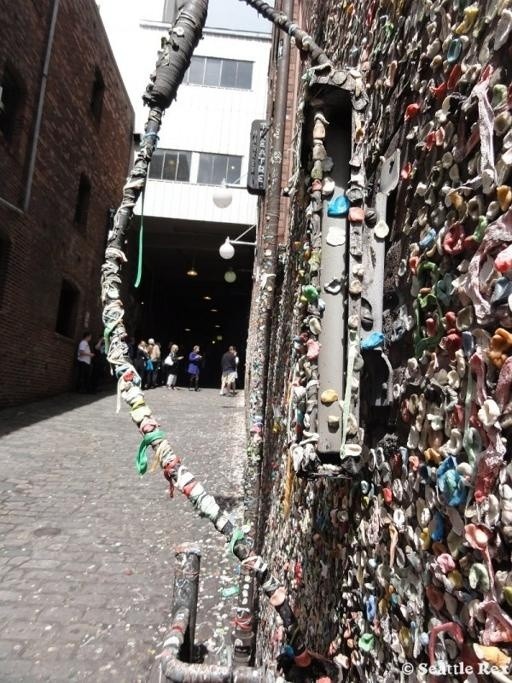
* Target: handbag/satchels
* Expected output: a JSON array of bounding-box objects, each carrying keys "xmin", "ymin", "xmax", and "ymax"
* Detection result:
[
  {"xmin": 164, "ymin": 352, "xmax": 174, "ymax": 366},
  {"xmin": 144, "ymin": 359, "xmax": 154, "ymax": 370}
]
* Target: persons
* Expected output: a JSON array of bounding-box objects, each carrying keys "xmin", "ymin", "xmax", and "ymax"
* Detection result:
[
  {"xmin": 219, "ymin": 346, "xmax": 239, "ymax": 397},
  {"xmin": 127, "ymin": 336, "xmax": 204, "ymax": 391},
  {"xmin": 75, "ymin": 331, "xmax": 105, "ymax": 394}
]
